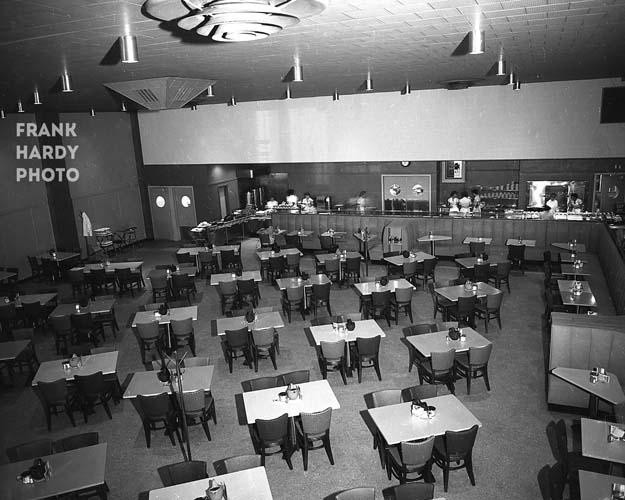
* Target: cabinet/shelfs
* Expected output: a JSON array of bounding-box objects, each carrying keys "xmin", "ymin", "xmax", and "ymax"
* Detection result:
[{"xmin": 468, "ymin": 189, "xmax": 519, "ymax": 217}]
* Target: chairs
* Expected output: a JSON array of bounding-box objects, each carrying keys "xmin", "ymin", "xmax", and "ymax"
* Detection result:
[{"xmin": 0, "ymin": 217, "xmax": 625, "ymax": 500}]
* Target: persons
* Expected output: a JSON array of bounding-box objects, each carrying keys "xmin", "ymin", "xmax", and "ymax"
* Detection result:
[
  {"xmin": 266, "ymin": 196, "xmax": 279, "ymax": 209},
  {"xmin": 471, "ymin": 190, "xmax": 482, "ymax": 216},
  {"xmin": 546, "ymin": 193, "xmax": 559, "ymax": 213},
  {"xmin": 355, "ymin": 191, "xmax": 366, "ymax": 215},
  {"xmin": 285, "ymin": 188, "xmax": 300, "ymax": 214},
  {"xmin": 459, "ymin": 191, "xmax": 472, "ymax": 218},
  {"xmin": 568, "ymin": 193, "xmax": 585, "ymax": 212},
  {"xmin": 302, "ymin": 192, "xmax": 315, "ymax": 207},
  {"xmin": 447, "ymin": 191, "xmax": 459, "ymax": 217}
]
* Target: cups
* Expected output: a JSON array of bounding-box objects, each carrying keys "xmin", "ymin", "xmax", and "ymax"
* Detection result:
[{"xmin": 279, "ymin": 392, "xmax": 288, "ymax": 401}]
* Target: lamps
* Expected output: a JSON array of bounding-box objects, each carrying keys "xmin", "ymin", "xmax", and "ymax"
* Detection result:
[
  {"xmin": 0, "ymin": 35, "xmax": 139, "ymax": 118},
  {"xmin": 190, "ymin": 66, "xmax": 411, "ymax": 112},
  {"xmin": 468, "ymin": 29, "xmax": 520, "ymax": 91}
]
